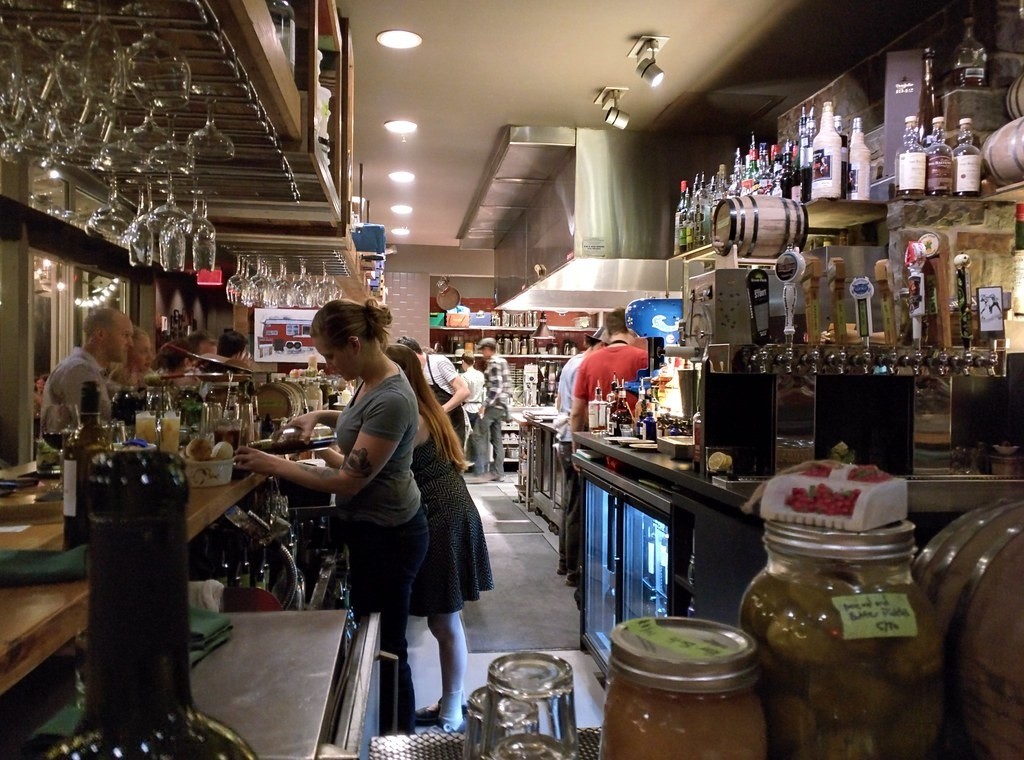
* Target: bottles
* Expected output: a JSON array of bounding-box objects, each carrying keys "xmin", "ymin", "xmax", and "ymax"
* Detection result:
[
  {"xmin": 672, "ymin": 101, "xmax": 871, "ymax": 252},
  {"xmin": 738, "ymin": 521, "xmax": 946, "ymax": 760},
  {"xmin": 953, "ymin": 117, "xmax": 982, "ymax": 197},
  {"xmin": 63, "ymin": 380, "xmax": 111, "ymax": 548},
  {"xmin": 251, "ymin": 370, "xmax": 353, "ymax": 451},
  {"xmin": 27, "ymin": 450, "xmax": 264, "ymax": 760},
  {"xmin": 692, "ymin": 406, "xmax": 700, "ymax": 473},
  {"xmin": 951, "ymin": 17, "xmax": 987, "ymax": 88},
  {"xmin": 599, "ymin": 616, "xmax": 767, "ymax": 760},
  {"xmin": 893, "ymin": 115, "xmax": 926, "ymax": 195},
  {"xmin": 925, "ymin": 117, "xmax": 954, "ymax": 196},
  {"xmin": 588, "ymin": 373, "xmax": 676, "ymax": 441}
]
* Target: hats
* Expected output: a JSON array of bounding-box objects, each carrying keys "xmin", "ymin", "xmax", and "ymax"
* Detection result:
[
  {"xmin": 477, "ymin": 337, "xmax": 497, "ymax": 349},
  {"xmin": 585, "ymin": 327, "xmax": 608, "ymax": 346}
]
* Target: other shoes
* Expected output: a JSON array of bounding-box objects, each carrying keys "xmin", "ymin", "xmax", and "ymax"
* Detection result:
[
  {"xmin": 565, "ymin": 570, "xmax": 578, "ymax": 587},
  {"xmin": 470, "ymin": 474, "xmax": 485, "ymax": 482},
  {"xmin": 557, "ymin": 560, "xmax": 567, "ymax": 575},
  {"xmin": 414, "ymin": 705, "xmax": 468, "ymax": 727},
  {"xmin": 437, "ymin": 717, "xmax": 466, "ymax": 735},
  {"xmin": 486, "ymin": 471, "xmax": 505, "ymax": 482}
]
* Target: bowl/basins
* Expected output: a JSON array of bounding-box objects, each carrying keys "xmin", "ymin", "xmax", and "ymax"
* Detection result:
[{"xmin": 184, "ymin": 457, "xmax": 234, "ymax": 488}]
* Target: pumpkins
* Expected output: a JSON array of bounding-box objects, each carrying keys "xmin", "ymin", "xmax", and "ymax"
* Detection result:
[
  {"xmin": 142, "ymin": 369, "xmax": 166, "ymax": 387},
  {"xmin": 182, "ymin": 438, "xmax": 236, "ymax": 462}
]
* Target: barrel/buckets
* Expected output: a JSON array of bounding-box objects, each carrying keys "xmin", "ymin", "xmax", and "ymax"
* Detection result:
[
  {"xmin": 911, "ymin": 498, "xmax": 1024, "ymax": 760},
  {"xmin": 712, "ymin": 195, "xmax": 808, "ymax": 260},
  {"xmin": 981, "ymin": 115, "xmax": 1024, "ymax": 184},
  {"xmin": 1006, "ymin": 73, "xmax": 1024, "ymax": 119}
]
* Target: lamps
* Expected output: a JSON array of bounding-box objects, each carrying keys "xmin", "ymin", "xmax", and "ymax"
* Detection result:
[
  {"xmin": 593, "ymin": 87, "xmax": 630, "ymax": 130},
  {"xmin": 628, "ymin": 35, "xmax": 670, "ymax": 87}
]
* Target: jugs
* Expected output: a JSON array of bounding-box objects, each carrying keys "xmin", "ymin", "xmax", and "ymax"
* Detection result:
[{"xmin": 494, "ymin": 311, "xmax": 578, "ymax": 356}]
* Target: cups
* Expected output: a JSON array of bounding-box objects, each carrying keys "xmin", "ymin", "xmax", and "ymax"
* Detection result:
[
  {"xmin": 463, "ymin": 653, "xmax": 581, "ymax": 760},
  {"xmin": 500, "ymin": 421, "xmax": 518, "ymax": 440},
  {"xmin": 136, "ymin": 410, "xmax": 181, "ymax": 456},
  {"xmin": 213, "ymin": 419, "xmax": 242, "ymax": 451}
]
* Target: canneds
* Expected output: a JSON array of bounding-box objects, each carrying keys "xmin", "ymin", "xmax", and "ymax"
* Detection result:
[{"xmin": 598, "ymin": 517, "xmax": 946, "ymax": 760}]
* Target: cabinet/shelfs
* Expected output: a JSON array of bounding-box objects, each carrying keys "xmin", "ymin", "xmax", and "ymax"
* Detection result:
[
  {"xmin": 429, "ymin": 326, "xmax": 598, "ymax": 462},
  {"xmin": 0, "ymin": 0, "xmax": 387, "ymax": 309},
  {"xmin": 488, "ymin": 406, "xmax": 558, "ymax": 462}
]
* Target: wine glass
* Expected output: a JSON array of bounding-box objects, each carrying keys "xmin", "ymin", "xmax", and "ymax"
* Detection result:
[
  {"xmin": 0, "ymin": 0, "xmax": 344, "ymax": 309},
  {"xmin": 40, "ymin": 402, "xmax": 80, "ymax": 493}
]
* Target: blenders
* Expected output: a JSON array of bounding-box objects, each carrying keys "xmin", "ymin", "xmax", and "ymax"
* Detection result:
[{"xmin": 539, "ymin": 359, "xmax": 558, "ymax": 405}]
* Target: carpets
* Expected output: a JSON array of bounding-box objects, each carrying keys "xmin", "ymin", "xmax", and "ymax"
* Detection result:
[
  {"xmin": 463, "ymin": 473, "xmax": 515, "ymax": 484},
  {"xmin": 466, "ymin": 485, "xmax": 545, "ymax": 534},
  {"xmin": 462, "ymin": 534, "xmax": 582, "ymax": 653}
]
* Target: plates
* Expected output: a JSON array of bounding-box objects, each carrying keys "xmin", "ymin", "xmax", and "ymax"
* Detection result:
[{"xmin": 605, "ymin": 437, "xmax": 659, "ymax": 450}]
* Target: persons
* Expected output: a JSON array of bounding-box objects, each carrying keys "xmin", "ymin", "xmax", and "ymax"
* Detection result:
[
  {"xmin": 33, "ymin": 306, "xmax": 248, "ymax": 438},
  {"xmin": 237, "ymin": 300, "xmax": 430, "ymax": 736},
  {"xmin": 294, "ymin": 336, "xmax": 493, "ymax": 734},
  {"xmin": 466, "ymin": 337, "xmax": 514, "ymax": 483},
  {"xmin": 557, "ymin": 305, "xmax": 649, "ymax": 611}
]
